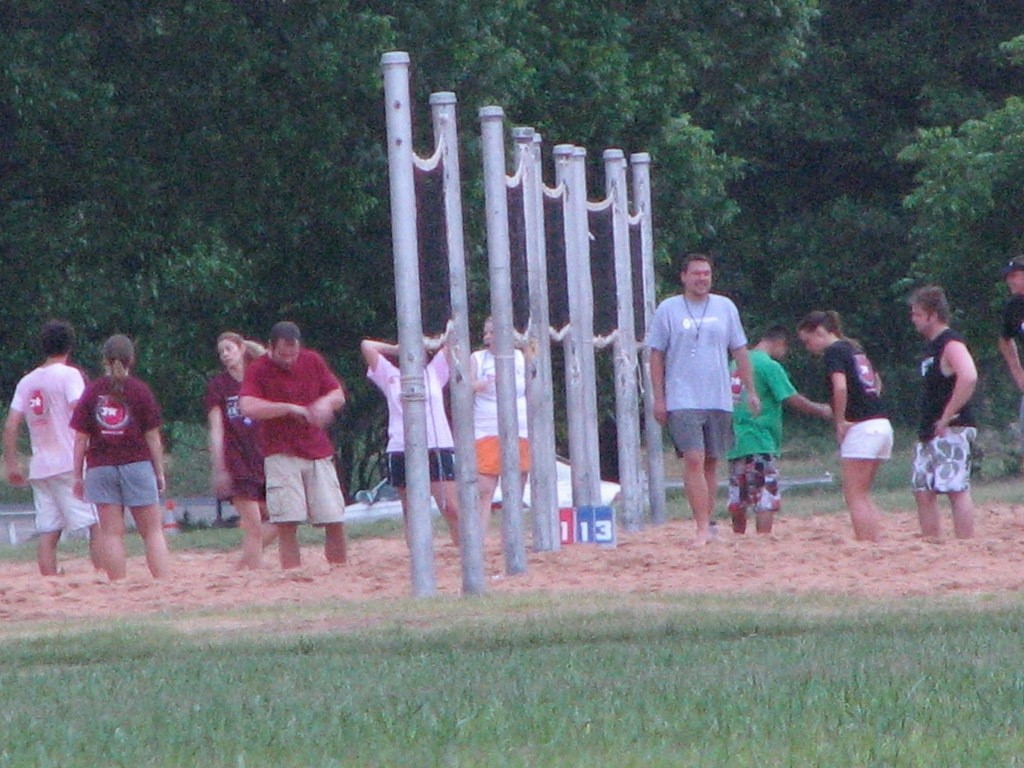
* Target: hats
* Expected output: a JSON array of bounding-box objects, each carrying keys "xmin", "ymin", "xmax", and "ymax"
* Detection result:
[{"xmin": 999, "ymin": 261, "xmax": 1024, "ymax": 273}]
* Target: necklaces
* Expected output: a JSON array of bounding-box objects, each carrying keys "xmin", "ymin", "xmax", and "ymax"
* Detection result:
[{"xmin": 684, "ymin": 293, "xmax": 710, "ymax": 343}]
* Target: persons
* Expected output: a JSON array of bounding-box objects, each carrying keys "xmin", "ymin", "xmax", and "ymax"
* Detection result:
[
  {"xmin": 906, "ymin": 284, "xmax": 978, "ymax": 543},
  {"xmin": 643, "ymin": 253, "xmax": 760, "ymax": 546},
  {"xmin": 725, "ymin": 324, "xmax": 835, "ymax": 536},
  {"xmin": 2, "ymin": 314, "xmax": 533, "ymax": 587},
  {"xmin": 996, "ymin": 254, "xmax": 1024, "ymax": 442},
  {"xmin": 796, "ymin": 310, "xmax": 894, "ymax": 545}
]
